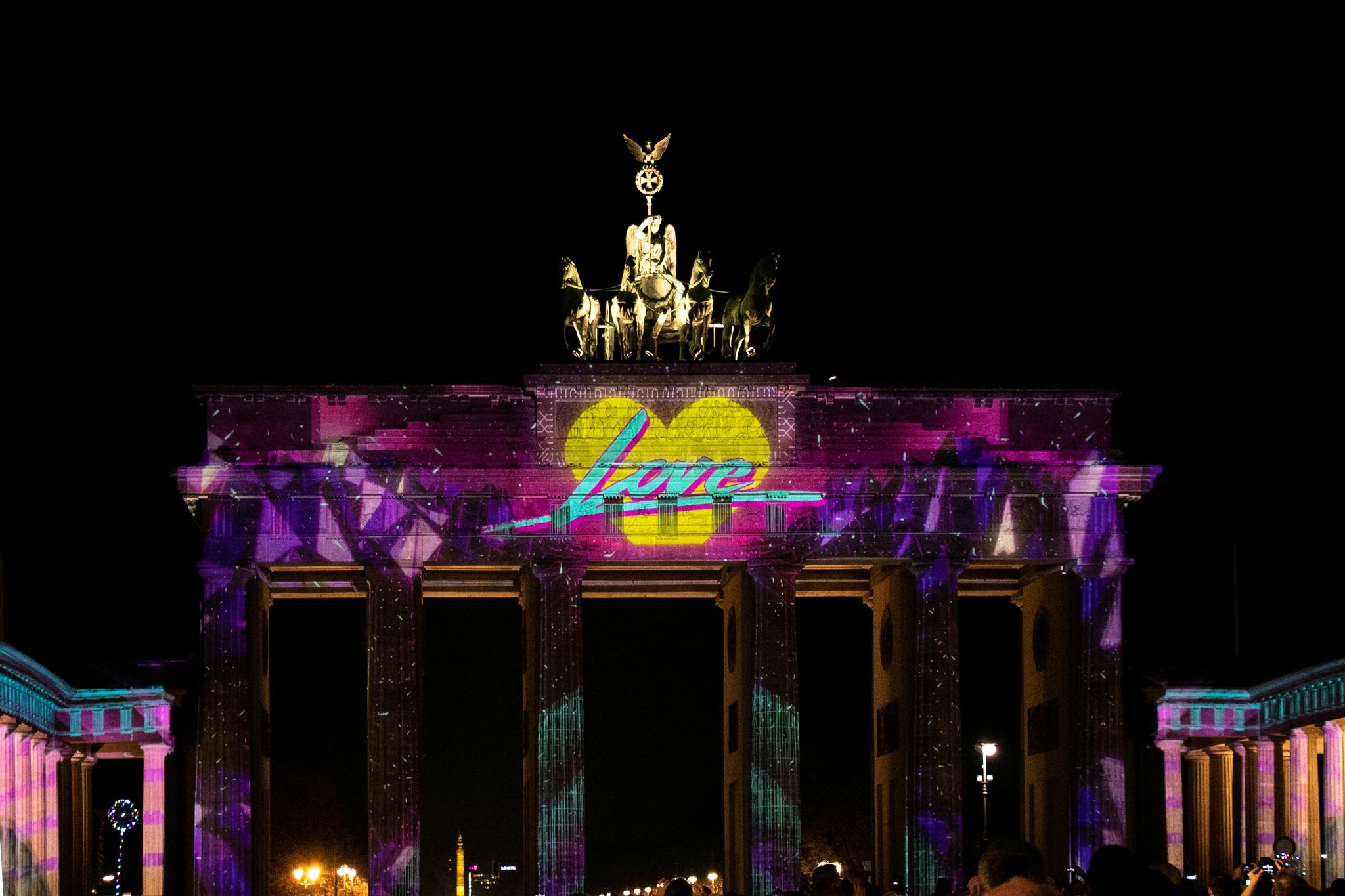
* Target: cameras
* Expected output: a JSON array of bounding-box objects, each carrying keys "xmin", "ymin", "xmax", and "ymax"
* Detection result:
[{"xmin": 1257, "ymin": 857, "xmax": 1282, "ymax": 877}]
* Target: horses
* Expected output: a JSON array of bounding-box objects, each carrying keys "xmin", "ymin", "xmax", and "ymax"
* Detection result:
[
  {"xmin": 722, "ymin": 252, "xmax": 779, "ymax": 361},
  {"xmin": 679, "ymin": 251, "xmax": 713, "ymax": 362},
  {"xmin": 557, "ymin": 256, "xmax": 599, "ymax": 360},
  {"xmin": 604, "ymin": 255, "xmax": 646, "ymax": 361}
]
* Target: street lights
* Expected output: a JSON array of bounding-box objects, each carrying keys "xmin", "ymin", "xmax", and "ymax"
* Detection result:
[
  {"xmin": 708, "ymin": 872, "xmax": 718, "ymax": 888},
  {"xmin": 977, "ymin": 742, "xmax": 1000, "ymax": 852},
  {"xmin": 293, "ymin": 866, "xmax": 320, "ymax": 896},
  {"xmin": 645, "ymin": 887, "xmax": 652, "ymax": 896},
  {"xmin": 331, "ymin": 863, "xmax": 356, "ymax": 896},
  {"xmin": 688, "ymin": 876, "xmax": 697, "ymax": 890}
]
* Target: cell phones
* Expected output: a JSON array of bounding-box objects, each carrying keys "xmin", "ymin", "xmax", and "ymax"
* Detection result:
[
  {"xmin": 1241, "ymin": 864, "xmax": 1254, "ymax": 874},
  {"xmin": 1186, "ymin": 874, "xmax": 1199, "ymax": 879},
  {"xmin": 1066, "ymin": 867, "xmax": 1076, "ymax": 872}
]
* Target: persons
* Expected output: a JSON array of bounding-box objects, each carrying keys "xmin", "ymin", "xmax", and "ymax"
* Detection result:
[
  {"xmin": 634, "ymin": 215, "xmax": 667, "ymax": 276},
  {"xmin": 663, "ymin": 839, "xmax": 1345, "ymax": 896}
]
